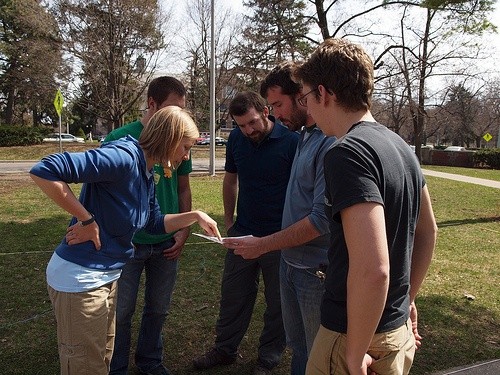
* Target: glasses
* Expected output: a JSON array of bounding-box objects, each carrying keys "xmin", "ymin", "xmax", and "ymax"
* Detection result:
[{"xmin": 297, "ymin": 83, "xmax": 334, "ymax": 107}]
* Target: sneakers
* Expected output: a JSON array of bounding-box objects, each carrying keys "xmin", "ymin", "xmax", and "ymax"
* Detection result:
[
  {"xmin": 192, "ymin": 348, "xmax": 235, "ymax": 370},
  {"xmin": 254, "ymin": 359, "xmax": 271, "ymax": 375}
]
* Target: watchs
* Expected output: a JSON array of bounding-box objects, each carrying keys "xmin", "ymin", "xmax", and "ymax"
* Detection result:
[{"xmin": 78, "ymin": 213, "xmax": 95, "ymax": 227}]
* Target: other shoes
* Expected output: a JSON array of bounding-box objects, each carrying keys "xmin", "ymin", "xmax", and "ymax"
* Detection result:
[{"xmin": 148, "ymin": 364, "xmax": 166, "ymax": 375}]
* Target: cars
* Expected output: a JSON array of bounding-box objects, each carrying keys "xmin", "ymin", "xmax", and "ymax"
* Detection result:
[
  {"xmin": 444, "ymin": 146, "xmax": 466, "ymax": 151},
  {"xmin": 197, "ymin": 138, "xmax": 228, "ymax": 146}
]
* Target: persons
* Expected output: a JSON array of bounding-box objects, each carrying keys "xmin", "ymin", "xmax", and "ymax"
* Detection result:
[
  {"xmin": 194, "ymin": 90, "xmax": 304, "ymax": 370},
  {"xmin": 290, "ymin": 39, "xmax": 441, "ymax": 375},
  {"xmin": 29, "ymin": 106, "xmax": 222, "ymax": 375},
  {"xmin": 103, "ymin": 76, "xmax": 193, "ymax": 375},
  {"xmin": 222, "ymin": 61, "xmax": 338, "ymax": 375}
]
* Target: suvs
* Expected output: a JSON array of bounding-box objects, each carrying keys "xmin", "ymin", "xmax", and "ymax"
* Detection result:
[{"xmin": 43, "ymin": 133, "xmax": 86, "ymax": 144}]
